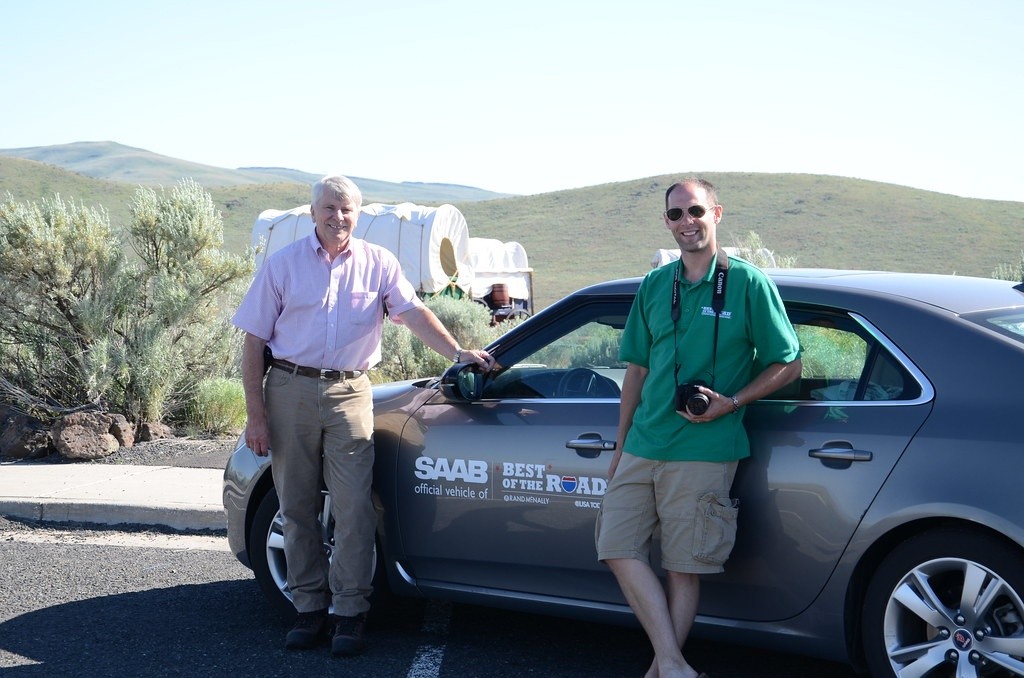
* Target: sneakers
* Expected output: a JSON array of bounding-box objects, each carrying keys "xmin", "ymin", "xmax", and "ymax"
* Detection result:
[
  {"xmin": 284, "ymin": 612, "xmax": 329, "ymax": 649},
  {"xmin": 331, "ymin": 615, "xmax": 368, "ymax": 655}
]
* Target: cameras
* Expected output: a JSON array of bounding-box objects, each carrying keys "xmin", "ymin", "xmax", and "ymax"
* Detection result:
[{"xmin": 674, "ymin": 379, "xmax": 713, "ymax": 415}]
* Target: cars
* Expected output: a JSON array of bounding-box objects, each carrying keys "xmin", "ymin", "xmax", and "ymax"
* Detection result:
[{"xmin": 222, "ymin": 268, "xmax": 1023, "ymax": 678}]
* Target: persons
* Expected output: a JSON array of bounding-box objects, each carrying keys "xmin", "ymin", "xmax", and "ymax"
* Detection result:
[
  {"xmin": 594, "ymin": 181, "xmax": 801, "ymax": 678},
  {"xmin": 234, "ymin": 176, "xmax": 505, "ymax": 656}
]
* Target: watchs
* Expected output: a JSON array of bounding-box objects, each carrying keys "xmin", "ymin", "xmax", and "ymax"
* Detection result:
[
  {"xmin": 453, "ymin": 348, "xmax": 462, "ymax": 363},
  {"xmin": 730, "ymin": 395, "xmax": 740, "ymax": 414}
]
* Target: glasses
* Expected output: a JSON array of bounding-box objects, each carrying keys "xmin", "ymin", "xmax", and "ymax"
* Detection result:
[{"xmin": 664, "ymin": 205, "xmax": 716, "ymax": 221}]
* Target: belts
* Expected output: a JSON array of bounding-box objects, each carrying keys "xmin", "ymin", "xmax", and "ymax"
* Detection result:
[{"xmin": 271, "ymin": 359, "xmax": 364, "ymax": 381}]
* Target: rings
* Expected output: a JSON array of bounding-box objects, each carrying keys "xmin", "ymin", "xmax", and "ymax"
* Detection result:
[{"xmin": 697, "ymin": 420, "xmax": 699, "ymax": 423}]
